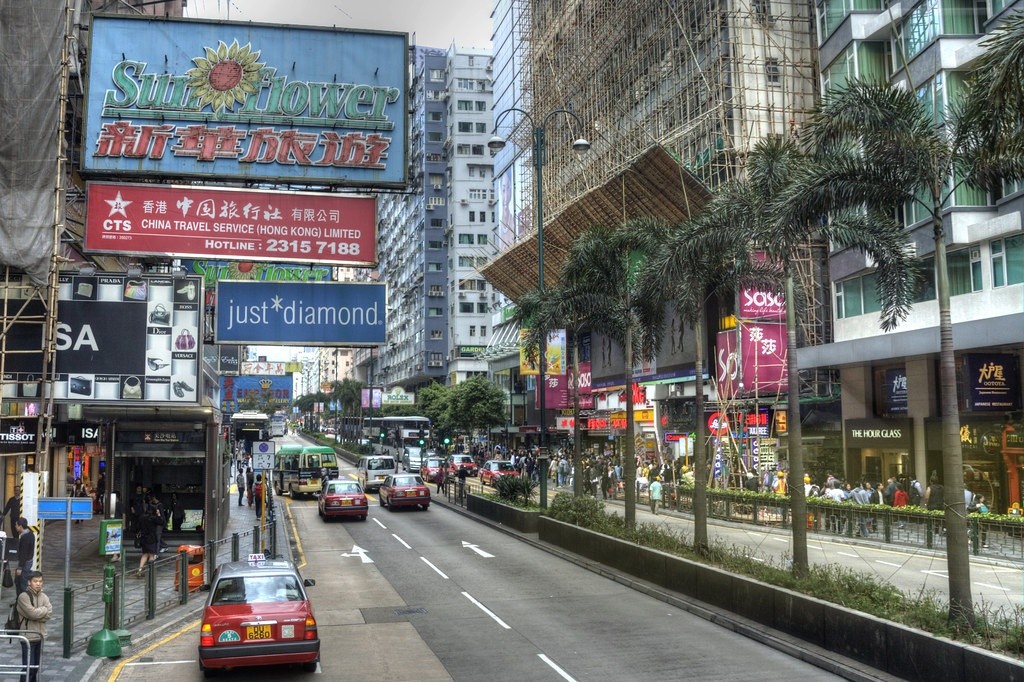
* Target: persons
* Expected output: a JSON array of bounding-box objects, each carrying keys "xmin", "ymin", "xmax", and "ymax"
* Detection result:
[
  {"xmin": 237, "ymin": 449, "xmax": 241, "ymax": 471},
  {"xmin": 805, "ymin": 468, "xmax": 885, "ymax": 538},
  {"xmin": 3, "ymin": 486, "xmax": 21, "ymax": 538},
  {"xmin": 456, "ymin": 464, "xmax": 470, "ymax": 498},
  {"xmin": 926, "ymin": 470, "xmax": 989, "ymax": 546},
  {"xmin": 127, "ymin": 485, "xmax": 169, "ymax": 575},
  {"xmin": 635, "ymin": 457, "xmax": 670, "ymax": 515},
  {"xmin": 237, "ymin": 466, "xmax": 263, "ymax": 520},
  {"xmin": 885, "ymin": 473, "xmax": 924, "ymax": 528},
  {"xmin": 744, "ymin": 466, "xmax": 759, "ymax": 494},
  {"xmin": 170, "ymin": 492, "xmax": 185, "ymax": 531},
  {"xmin": 773, "ymin": 470, "xmax": 790, "ymax": 523},
  {"xmin": 70, "ymin": 477, "xmax": 88, "ymax": 524},
  {"xmin": 454, "ymin": 441, "xmax": 492, "ymax": 461},
  {"xmin": 16, "ymin": 571, "xmax": 53, "ymax": 682},
  {"xmin": 67, "ymin": 467, "xmax": 74, "ymax": 483},
  {"xmin": 436, "ymin": 464, "xmax": 445, "ymax": 494},
  {"xmin": 14, "ymin": 517, "xmax": 35, "ymax": 601},
  {"xmin": 93, "ymin": 473, "xmax": 105, "ymax": 515},
  {"xmin": 493, "ymin": 441, "xmax": 622, "ymax": 499}
]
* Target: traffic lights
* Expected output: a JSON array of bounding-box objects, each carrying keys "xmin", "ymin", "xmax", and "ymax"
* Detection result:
[
  {"xmin": 433, "ymin": 428, "xmax": 444, "ymax": 444},
  {"xmin": 391, "ymin": 428, "xmax": 400, "ymax": 442},
  {"xmin": 380, "ymin": 426, "xmax": 387, "ymax": 440},
  {"xmin": 444, "ymin": 425, "xmax": 453, "ymax": 445},
  {"xmin": 500, "ymin": 428, "xmax": 507, "ymax": 439},
  {"xmin": 419, "ymin": 429, "xmax": 426, "ymax": 448}
]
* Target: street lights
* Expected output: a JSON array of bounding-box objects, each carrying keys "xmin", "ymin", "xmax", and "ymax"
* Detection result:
[{"xmin": 486, "ymin": 106, "xmax": 594, "ymax": 503}]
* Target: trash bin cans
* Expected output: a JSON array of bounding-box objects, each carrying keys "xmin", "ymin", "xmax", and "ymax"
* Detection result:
[
  {"xmin": 174, "ymin": 545, "xmax": 204, "ymax": 592},
  {"xmin": 807, "ymin": 512, "xmax": 822, "ymax": 528}
]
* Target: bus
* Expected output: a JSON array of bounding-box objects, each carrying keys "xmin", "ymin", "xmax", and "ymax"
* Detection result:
[
  {"xmin": 271, "ymin": 415, "xmax": 286, "ymax": 436},
  {"xmin": 228, "ymin": 410, "xmax": 273, "ymax": 455},
  {"xmin": 340, "ymin": 416, "xmax": 431, "ymax": 461},
  {"xmin": 271, "ymin": 444, "xmax": 340, "ymax": 500}
]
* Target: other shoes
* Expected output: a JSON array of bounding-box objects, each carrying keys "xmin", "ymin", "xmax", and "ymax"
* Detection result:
[
  {"xmin": 239, "ymin": 504, "xmax": 244, "ymax": 506},
  {"xmin": 982, "ymin": 545, "xmax": 988, "ymax": 547}
]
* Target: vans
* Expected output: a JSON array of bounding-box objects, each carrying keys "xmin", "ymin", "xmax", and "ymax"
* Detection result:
[
  {"xmin": 355, "ymin": 456, "xmax": 397, "ymax": 493},
  {"xmin": 402, "ymin": 447, "xmax": 428, "ymax": 472}
]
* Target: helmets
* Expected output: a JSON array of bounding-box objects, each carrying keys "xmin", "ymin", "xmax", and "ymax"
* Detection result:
[
  {"xmin": 804, "ymin": 477, "xmax": 810, "ymax": 484},
  {"xmin": 682, "ymin": 466, "xmax": 688, "ymax": 472},
  {"xmin": 778, "ymin": 472, "xmax": 783, "ymax": 477}
]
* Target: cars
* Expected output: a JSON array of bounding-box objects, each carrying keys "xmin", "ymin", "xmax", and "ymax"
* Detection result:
[
  {"xmin": 198, "ymin": 555, "xmax": 321, "ymax": 679},
  {"xmin": 420, "ymin": 458, "xmax": 460, "ymax": 481},
  {"xmin": 315, "ymin": 480, "xmax": 376, "ymax": 520},
  {"xmin": 448, "ymin": 455, "xmax": 479, "ymax": 475},
  {"xmin": 379, "ymin": 474, "xmax": 432, "ymax": 511},
  {"xmin": 480, "ymin": 461, "xmax": 522, "ymax": 483}
]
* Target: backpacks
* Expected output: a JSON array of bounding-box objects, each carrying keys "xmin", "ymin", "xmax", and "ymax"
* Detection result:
[
  {"xmin": 4, "ymin": 591, "xmax": 33, "ymax": 636},
  {"xmin": 255, "ymin": 482, "xmax": 261, "ymax": 498}
]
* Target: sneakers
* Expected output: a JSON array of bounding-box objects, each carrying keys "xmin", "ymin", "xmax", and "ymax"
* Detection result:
[{"xmin": 160, "ymin": 547, "xmax": 168, "ymax": 553}]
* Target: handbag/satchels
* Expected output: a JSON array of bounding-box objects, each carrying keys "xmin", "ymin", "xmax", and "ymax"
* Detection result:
[
  {"xmin": 134, "ymin": 535, "xmax": 141, "ymax": 548},
  {"xmin": 2, "ymin": 561, "xmax": 13, "ymax": 588}
]
